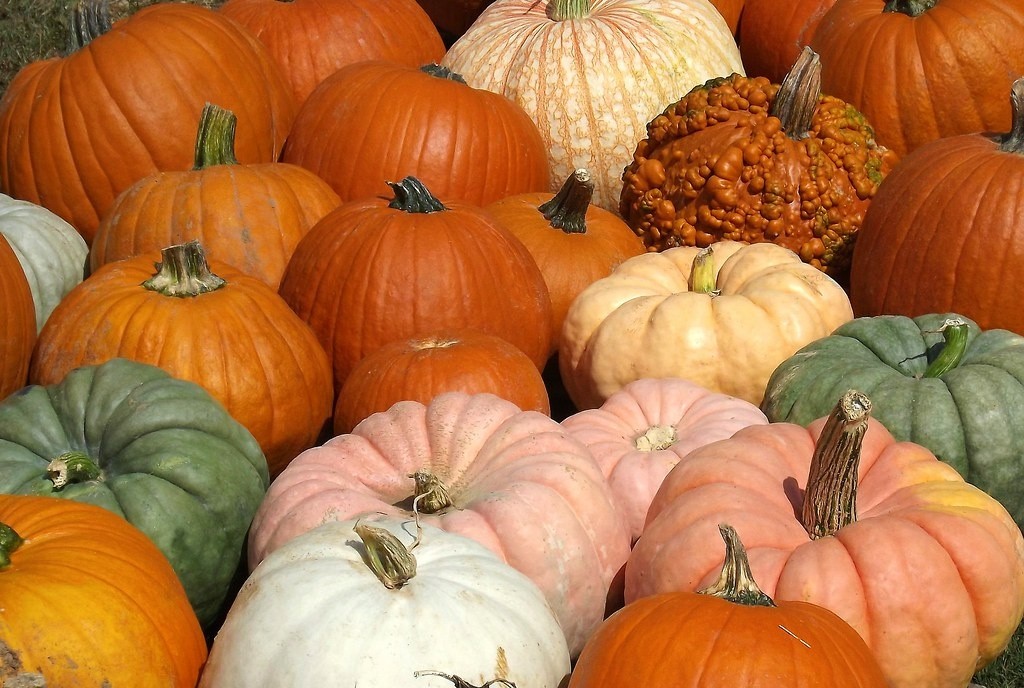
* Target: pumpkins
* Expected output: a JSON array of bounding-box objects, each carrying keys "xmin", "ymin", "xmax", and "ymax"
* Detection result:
[{"xmin": 0, "ymin": 0, "xmax": 1024, "ymax": 688}]
